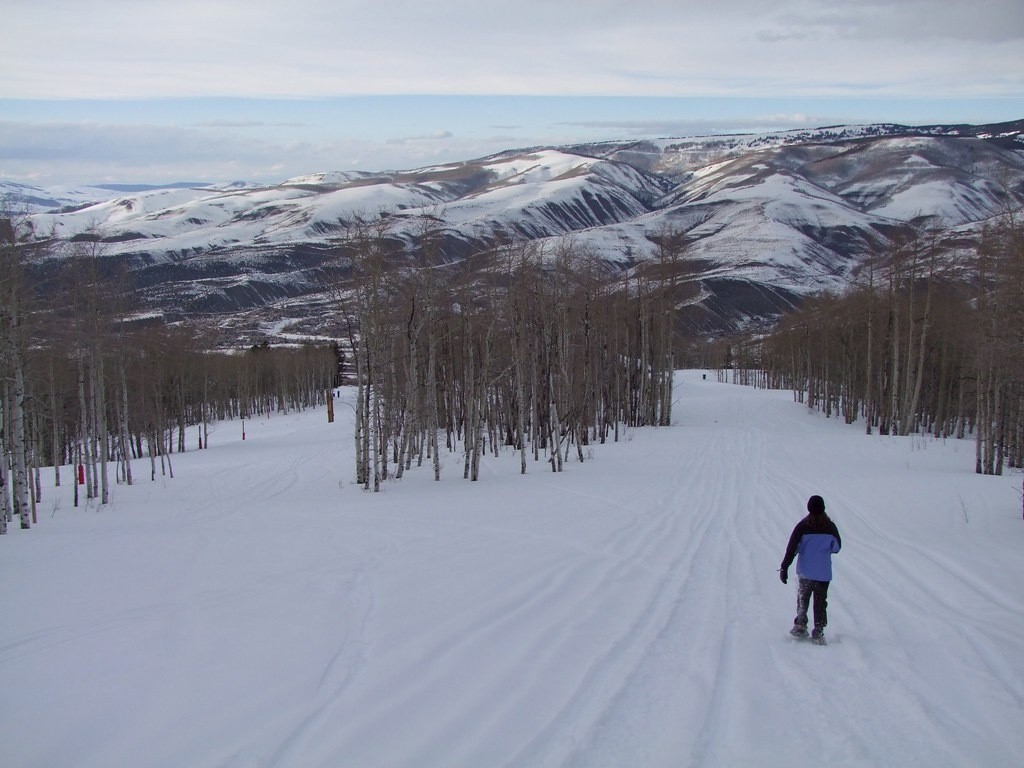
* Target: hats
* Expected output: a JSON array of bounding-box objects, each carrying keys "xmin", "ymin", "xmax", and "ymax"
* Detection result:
[{"xmin": 807, "ymin": 495, "xmax": 829, "ymax": 513}]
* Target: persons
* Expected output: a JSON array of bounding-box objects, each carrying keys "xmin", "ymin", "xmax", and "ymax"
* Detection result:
[{"xmin": 780, "ymin": 495, "xmax": 841, "ymax": 645}]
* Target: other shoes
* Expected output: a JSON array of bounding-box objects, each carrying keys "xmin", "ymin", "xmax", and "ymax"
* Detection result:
[
  {"xmin": 790, "ymin": 626, "xmax": 810, "ymax": 638},
  {"xmin": 814, "ymin": 628, "xmax": 826, "ymax": 642}
]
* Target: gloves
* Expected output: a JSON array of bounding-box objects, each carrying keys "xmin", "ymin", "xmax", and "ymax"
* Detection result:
[{"xmin": 779, "ymin": 569, "xmax": 792, "ymax": 585}]
